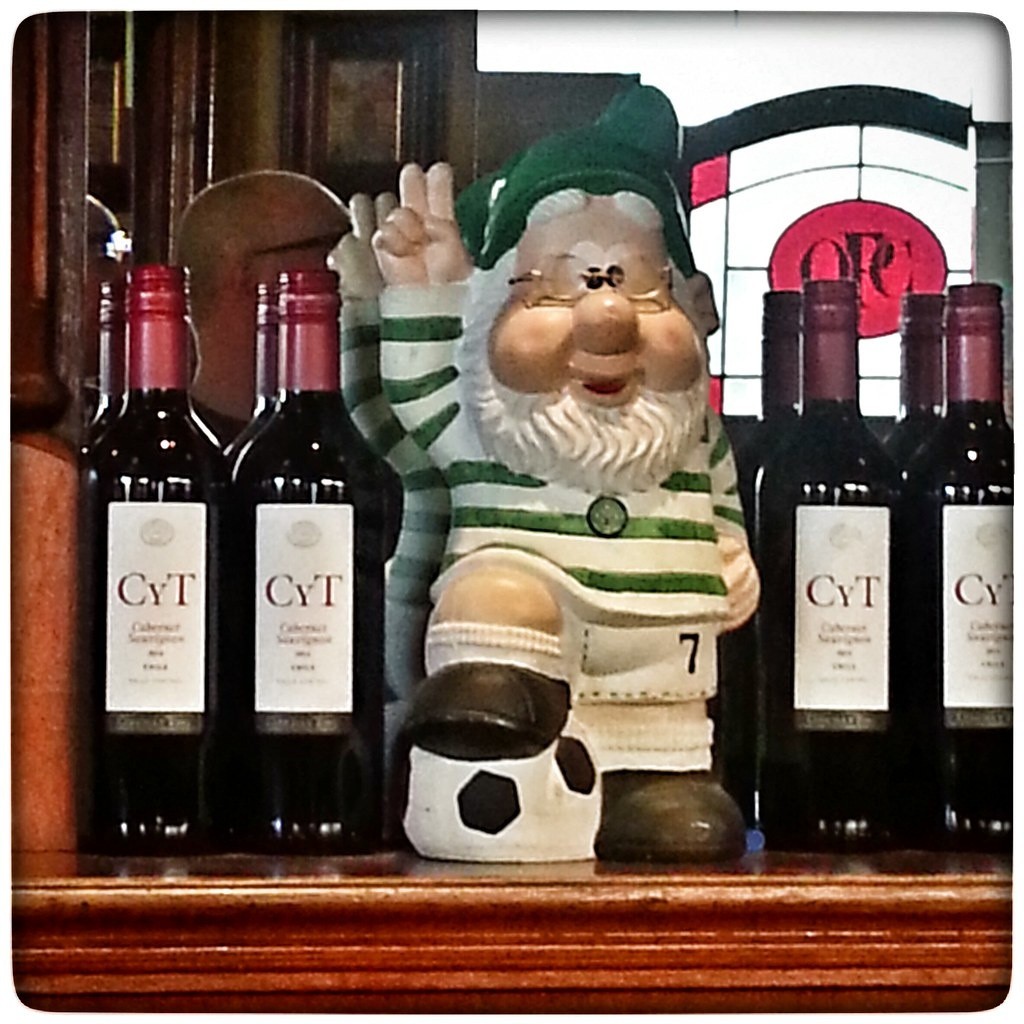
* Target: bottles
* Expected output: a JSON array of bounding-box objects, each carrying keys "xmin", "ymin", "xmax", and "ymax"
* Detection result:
[
  {"xmin": 223, "ymin": 282, "xmax": 281, "ymax": 456},
  {"xmin": 230, "ymin": 269, "xmax": 394, "ymax": 854},
  {"xmin": 902, "ymin": 285, "xmax": 1013, "ymax": 861},
  {"xmin": 718, "ymin": 290, "xmax": 805, "ymax": 821},
  {"xmin": 80, "ymin": 279, "xmax": 127, "ymax": 470},
  {"xmin": 85, "ymin": 270, "xmax": 231, "ymax": 855},
  {"xmin": 876, "ymin": 292, "xmax": 950, "ymax": 471},
  {"xmin": 753, "ymin": 279, "xmax": 901, "ymax": 857}
]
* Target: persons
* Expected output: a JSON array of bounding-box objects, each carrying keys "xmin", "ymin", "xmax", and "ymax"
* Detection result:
[
  {"xmin": 367, "ymin": 84, "xmax": 760, "ymax": 865},
  {"xmin": 325, "ymin": 193, "xmax": 452, "ymax": 697}
]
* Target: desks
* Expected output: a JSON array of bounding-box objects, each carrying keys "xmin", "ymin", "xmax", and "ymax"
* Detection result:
[{"xmin": 12, "ymin": 848, "xmax": 1013, "ymax": 1013}]
